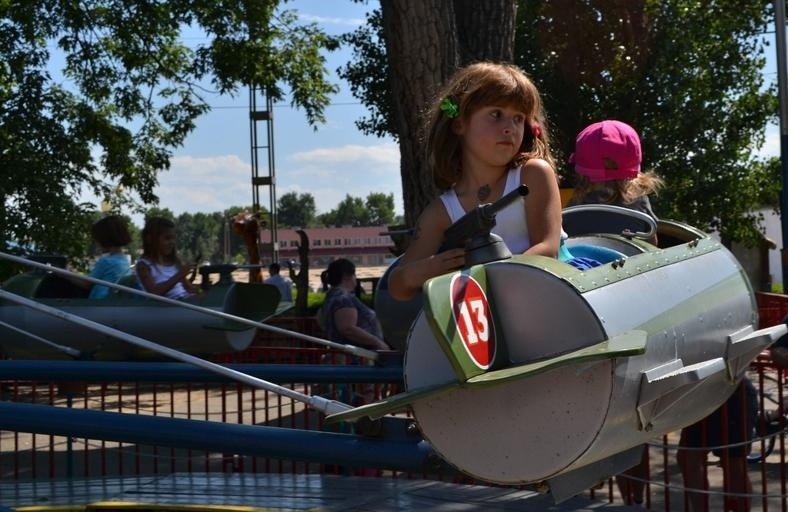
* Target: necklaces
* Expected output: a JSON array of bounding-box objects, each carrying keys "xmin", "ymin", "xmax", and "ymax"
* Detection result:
[{"xmin": 454, "ymin": 181, "xmax": 504, "ymax": 200}]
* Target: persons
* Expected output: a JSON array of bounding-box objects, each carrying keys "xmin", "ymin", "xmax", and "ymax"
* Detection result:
[
  {"xmin": 264, "ymin": 262, "xmax": 293, "ymax": 304},
  {"xmin": 316, "ymin": 257, "xmax": 392, "ymax": 438},
  {"xmin": 564, "ymin": 118, "xmax": 663, "ymax": 248},
  {"xmin": 613, "ymin": 444, "xmax": 649, "ymax": 505},
  {"xmin": 49, "ymin": 215, "xmax": 133, "ymax": 300},
  {"xmin": 134, "ymin": 215, "xmax": 203, "ymax": 303},
  {"xmin": 384, "ymin": 60, "xmax": 604, "ymax": 302},
  {"xmin": 675, "ymin": 376, "xmax": 760, "ymax": 511}
]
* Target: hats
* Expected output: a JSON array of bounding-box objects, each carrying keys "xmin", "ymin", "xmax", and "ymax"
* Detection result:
[{"xmin": 568, "ymin": 120, "xmax": 642, "ymax": 181}]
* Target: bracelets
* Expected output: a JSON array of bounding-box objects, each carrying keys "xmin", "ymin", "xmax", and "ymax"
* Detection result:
[{"xmin": 428, "ymin": 254, "xmax": 437, "ymax": 274}]
